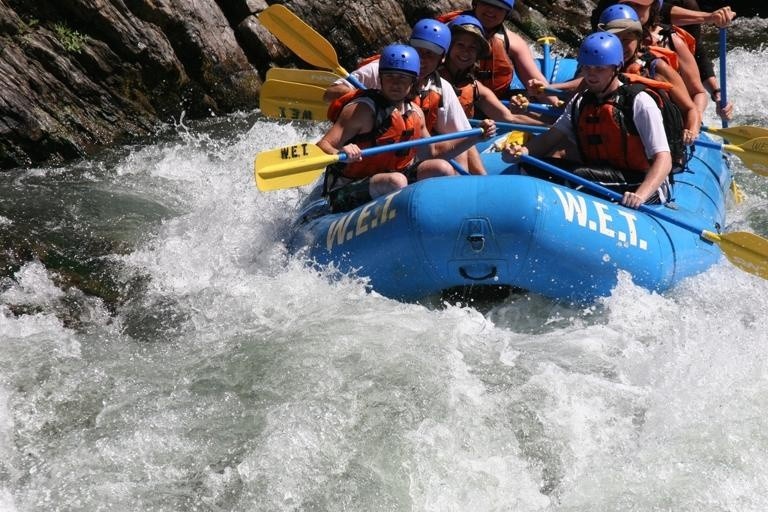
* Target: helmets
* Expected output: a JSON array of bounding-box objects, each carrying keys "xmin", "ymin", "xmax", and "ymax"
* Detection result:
[
  {"xmin": 577, "ymin": 32, "xmax": 625, "ymax": 71},
  {"xmin": 597, "ymin": 4, "xmax": 640, "ymax": 22},
  {"xmin": 378, "ymin": 44, "xmax": 421, "ymax": 82},
  {"xmin": 448, "ymin": 14, "xmax": 485, "ymax": 35},
  {"xmin": 409, "ymin": 17, "xmax": 453, "ymax": 59},
  {"xmin": 472, "ymin": 0, "xmax": 514, "ymax": 18}
]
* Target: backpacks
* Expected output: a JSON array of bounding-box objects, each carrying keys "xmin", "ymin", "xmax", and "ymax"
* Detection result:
[{"xmin": 570, "ymin": 84, "xmax": 694, "ymax": 183}]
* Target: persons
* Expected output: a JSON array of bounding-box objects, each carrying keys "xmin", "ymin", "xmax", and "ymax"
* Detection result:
[
  {"xmin": 320, "ymin": 19, "xmax": 487, "ymax": 176},
  {"xmin": 437, "ymin": 0, "xmax": 566, "ymax": 107},
  {"xmin": 318, "ymin": 45, "xmax": 497, "ymax": 212},
  {"xmin": 438, "ymin": 14, "xmax": 546, "ymax": 126},
  {"xmin": 506, "ymin": 30, "xmax": 673, "ymax": 210},
  {"xmin": 509, "ymin": 0, "xmax": 740, "ymax": 173}
]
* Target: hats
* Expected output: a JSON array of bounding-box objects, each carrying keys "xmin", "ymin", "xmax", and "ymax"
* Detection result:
[
  {"xmin": 452, "ymin": 24, "xmax": 492, "ymax": 57},
  {"xmin": 597, "ymin": 20, "xmax": 644, "ymax": 37}
]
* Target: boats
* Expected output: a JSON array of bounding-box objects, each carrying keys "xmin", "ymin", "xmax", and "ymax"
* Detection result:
[{"xmin": 284, "ymin": 53, "xmax": 733, "ymax": 313}]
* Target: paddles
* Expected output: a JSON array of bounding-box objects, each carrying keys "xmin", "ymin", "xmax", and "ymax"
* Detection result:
[
  {"xmin": 511, "ymin": 141, "xmax": 768, "ymax": 280},
  {"xmin": 719, "ymin": 28, "xmax": 741, "ymax": 205},
  {"xmin": 259, "ymin": 4, "xmax": 552, "ymax": 133},
  {"xmin": 501, "ymin": 35, "xmax": 556, "ymax": 151},
  {"xmin": 255, "ymin": 124, "xmax": 488, "ymax": 191},
  {"xmin": 512, "ymin": 80, "xmax": 768, "ymax": 177}
]
